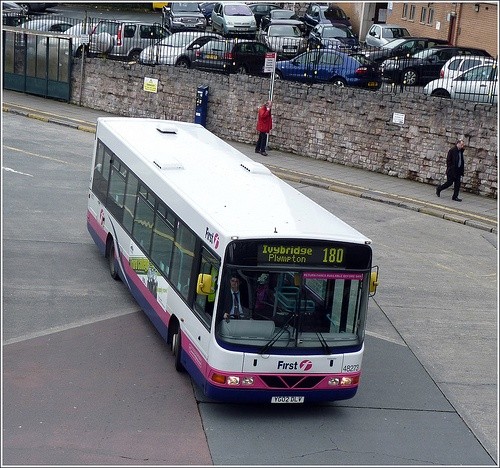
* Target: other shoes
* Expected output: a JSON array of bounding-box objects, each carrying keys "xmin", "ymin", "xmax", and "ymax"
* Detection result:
[
  {"xmin": 452, "ymin": 197, "xmax": 462, "ymax": 202},
  {"xmin": 436, "ymin": 187, "xmax": 441, "ymax": 197},
  {"xmin": 255, "ymin": 150, "xmax": 261, "ymax": 153},
  {"xmin": 261, "ymin": 152, "xmax": 268, "ymax": 156}
]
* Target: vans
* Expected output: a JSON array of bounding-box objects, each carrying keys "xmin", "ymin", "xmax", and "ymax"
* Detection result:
[{"xmin": 210, "ymin": 3, "xmax": 258, "ymax": 38}]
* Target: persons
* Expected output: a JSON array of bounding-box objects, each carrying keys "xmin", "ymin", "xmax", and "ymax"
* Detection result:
[
  {"xmin": 219, "ymin": 274, "xmax": 250, "ymax": 320},
  {"xmin": 255, "ymin": 100, "xmax": 273, "ymax": 156},
  {"xmin": 435, "ymin": 139, "xmax": 466, "ymax": 201}
]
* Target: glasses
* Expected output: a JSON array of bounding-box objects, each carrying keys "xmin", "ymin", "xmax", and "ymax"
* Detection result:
[{"xmin": 231, "ymin": 279, "xmax": 240, "ymax": 283}]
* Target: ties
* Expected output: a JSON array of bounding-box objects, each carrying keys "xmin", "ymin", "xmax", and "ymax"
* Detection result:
[{"xmin": 233, "ymin": 292, "xmax": 240, "ymax": 319}]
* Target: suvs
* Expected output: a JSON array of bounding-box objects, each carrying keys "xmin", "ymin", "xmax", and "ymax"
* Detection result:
[
  {"xmin": 162, "ymin": 2, "xmax": 207, "ymax": 32},
  {"xmin": 87, "ymin": 20, "xmax": 172, "ymax": 61}
]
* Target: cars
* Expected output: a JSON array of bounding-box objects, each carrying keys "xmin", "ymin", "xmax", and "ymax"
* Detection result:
[
  {"xmin": 2, "ymin": 2, "xmax": 104, "ymax": 57},
  {"xmin": 423, "ymin": 63, "xmax": 498, "ymax": 104},
  {"xmin": 200, "ymin": 3, "xmax": 363, "ymax": 56},
  {"xmin": 192, "ymin": 39, "xmax": 290, "ymax": 75},
  {"xmin": 347, "ymin": 24, "xmax": 496, "ymax": 85},
  {"xmin": 260, "ymin": 47, "xmax": 382, "ymax": 92},
  {"xmin": 139, "ymin": 31, "xmax": 225, "ymax": 68}
]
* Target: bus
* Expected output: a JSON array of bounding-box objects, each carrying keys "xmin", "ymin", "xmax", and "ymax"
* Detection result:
[{"xmin": 87, "ymin": 118, "xmax": 379, "ymax": 407}]
